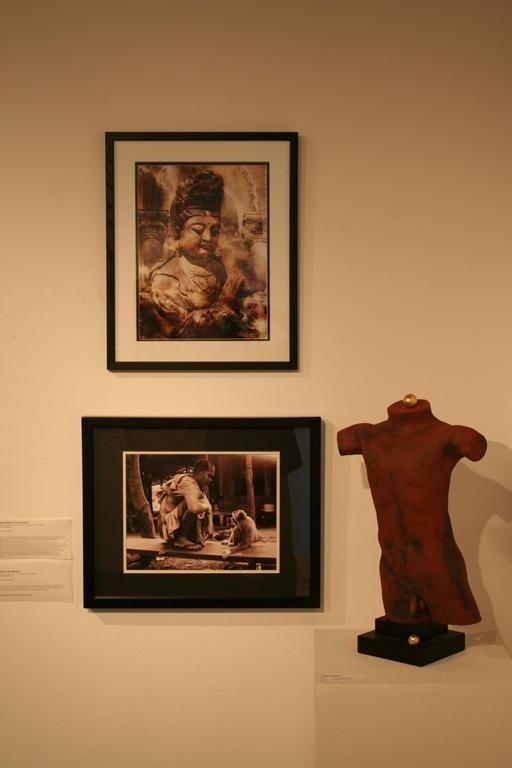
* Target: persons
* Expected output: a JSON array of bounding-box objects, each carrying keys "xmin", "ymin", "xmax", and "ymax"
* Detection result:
[
  {"xmin": 337, "ymin": 399, "xmax": 487, "ymax": 625},
  {"xmin": 150, "ymin": 171, "xmax": 245, "ymax": 338},
  {"xmin": 156, "ymin": 459, "xmax": 215, "ymax": 551}
]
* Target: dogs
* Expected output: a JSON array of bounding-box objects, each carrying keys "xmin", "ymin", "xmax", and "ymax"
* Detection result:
[{"xmin": 221, "ymin": 509, "xmax": 257, "ymax": 560}]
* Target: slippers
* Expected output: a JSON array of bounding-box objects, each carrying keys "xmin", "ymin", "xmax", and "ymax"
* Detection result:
[{"xmin": 176, "ymin": 544, "xmax": 201, "ymax": 551}]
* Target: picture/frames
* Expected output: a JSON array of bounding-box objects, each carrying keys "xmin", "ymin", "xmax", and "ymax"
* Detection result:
[
  {"xmin": 82, "ymin": 418, "xmax": 321, "ymax": 608},
  {"xmin": 106, "ymin": 132, "xmax": 298, "ymax": 370}
]
